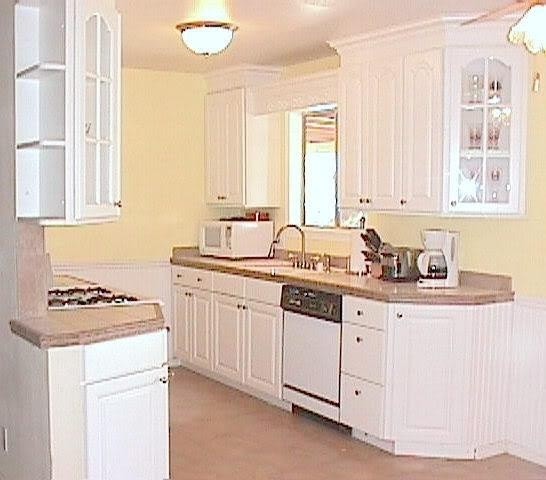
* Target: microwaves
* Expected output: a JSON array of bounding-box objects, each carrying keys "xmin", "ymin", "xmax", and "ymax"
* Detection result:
[{"xmin": 198, "ymin": 220, "xmax": 275, "ymax": 261}]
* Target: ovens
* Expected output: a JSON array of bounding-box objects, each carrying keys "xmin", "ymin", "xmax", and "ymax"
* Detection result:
[{"xmin": 280, "ymin": 284, "xmax": 343, "ymax": 407}]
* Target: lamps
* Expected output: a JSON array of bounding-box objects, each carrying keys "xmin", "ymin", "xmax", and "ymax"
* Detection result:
[{"xmin": 176, "ymin": 22, "xmax": 239, "ymax": 59}]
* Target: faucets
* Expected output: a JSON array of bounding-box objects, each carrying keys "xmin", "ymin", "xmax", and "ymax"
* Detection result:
[{"xmin": 273, "ymin": 224, "xmax": 306, "ymax": 269}]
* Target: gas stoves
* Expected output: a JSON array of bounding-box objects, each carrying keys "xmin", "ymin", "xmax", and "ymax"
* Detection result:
[{"xmin": 48, "ymin": 284, "xmax": 141, "ymax": 308}]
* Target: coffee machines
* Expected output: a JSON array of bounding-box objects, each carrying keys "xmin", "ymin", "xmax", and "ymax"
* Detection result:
[{"xmin": 416, "ymin": 228, "xmax": 461, "ymax": 289}]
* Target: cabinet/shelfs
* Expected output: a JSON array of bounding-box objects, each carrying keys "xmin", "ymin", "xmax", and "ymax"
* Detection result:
[
  {"xmin": 337, "ymin": 46, "xmax": 529, "ymax": 219},
  {"xmin": 14, "ymin": 0, "xmax": 122, "ymax": 226},
  {"xmin": 205, "ymin": 87, "xmax": 281, "ymax": 209},
  {"xmin": 85, "ymin": 328, "xmax": 169, "ymax": 480},
  {"xmin": 172, "ymin": 265, "xmax": 282, "ymax": 400},
  {"xmin": 339, "ymin": 295, "xmax": 514, "ymax": 458}
]
